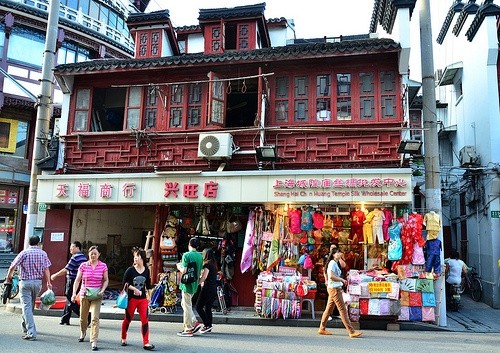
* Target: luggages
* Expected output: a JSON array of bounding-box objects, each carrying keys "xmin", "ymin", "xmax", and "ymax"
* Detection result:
[{"xmin": 210, "ymin": 280, "xmax": 232, "ymax": 311}]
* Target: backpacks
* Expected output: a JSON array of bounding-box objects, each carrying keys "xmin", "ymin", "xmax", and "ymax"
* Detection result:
[
  {"xmin": 297, "ymin": 253, "xmax": 315, "ymax": 269},
  {"xmin": 149, "ymin": 275, "xmax": 181, "ymax": 308},
  {"xmin": 197, "ymin": 216, "xmax": 211, "ymax": 235}
]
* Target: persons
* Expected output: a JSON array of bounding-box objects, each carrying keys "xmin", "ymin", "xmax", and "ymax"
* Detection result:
[
  {"xmin": 173, "ymin": 239, "xmax": 204, "ymax": 337},
  {"xmin": 443, "ymin": 251, "xmax": 467, "ymax": 311},
  {"xmin": 71, "ymin": 246, "xmax": 110, "ymax": 351},
  {"xmin": 121, "ymin": 249, "xmax": 155, "ymax": 350},
  {"xmin": 318, "ymin": 248, "xmax": 364, "ymax": 337},
  {"xmin": 51, "ymin": 241, "xmax": 91, "ymax": 327},
  {"xmin": 194, "ymin": 248, "xmax": 217, "ymax": 334},
  {"xmin": 323, "ymin": 242, "xmax": 348, "ymax": 321},
  {"xmin": 6, "ymin": 236, "xmax": 52, "ymax": 340}
]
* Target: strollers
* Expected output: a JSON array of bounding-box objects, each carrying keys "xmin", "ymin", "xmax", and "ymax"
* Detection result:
[{"xmin": 148, "ymin": 270, "xmax": 177, "ymax": 314}]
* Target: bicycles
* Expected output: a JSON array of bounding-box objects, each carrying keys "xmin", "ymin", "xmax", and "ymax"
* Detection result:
[
  {"xmin": 4, "ymin": 258, "xmax": 19, "ymax": 299},
  {"xmin": 459, "ymin": 267, "xmax": 483, "ymax": 302}
]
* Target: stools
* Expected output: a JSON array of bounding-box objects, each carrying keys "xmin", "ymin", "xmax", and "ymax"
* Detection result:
[{"xmin": 303, "ymin": 298, "xmax": 316, "ymax": 319}]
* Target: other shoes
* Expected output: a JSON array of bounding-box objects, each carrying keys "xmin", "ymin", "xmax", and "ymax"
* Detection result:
[
  {"xmin": 143, "ymin": 343, "xmax": 155, "ymax": 350},
  {"xmin": 349, "ymin": 331, "xmax": 363, "ymax": 336},
  {"xmin": 91, "ymin": 342, "xmax": 98, "ymax": 350},
  {"xmin": 87, "ymin": 312, "xmax": 91, "ymax": 325},
  {"xmin": 319, "ymin": 331, "xmax": 332, "ymax": 335},
  {"xmin": 78, "ymin": 332, "xmax": 87, "ymax": 342},
  {"xmin": 22, "ymin": 334, "xmax": 36, "ymax": 341},
  {"xmin": 121, "ymin": 338, "xmax": 127, "ymax": 346}
]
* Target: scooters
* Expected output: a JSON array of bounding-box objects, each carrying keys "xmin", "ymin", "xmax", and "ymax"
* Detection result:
[{"xmin": 446, "ymin": 281, "xmax": 463, "ymax": 312}]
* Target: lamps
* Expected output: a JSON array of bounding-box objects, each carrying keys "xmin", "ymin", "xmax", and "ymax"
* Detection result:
[
  {"xmin": 256, "ymin": 146, "xmax": 277, "ymax": 161},
  {"xmin": 397, "ymin": 139, "xmax": 423, "ymax": 168}
]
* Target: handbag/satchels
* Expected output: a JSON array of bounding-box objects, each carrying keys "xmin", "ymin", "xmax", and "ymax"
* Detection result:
[
  {"xmin": 333, "ymin": 281, "xmax": 343, "ymax": 289},
  {"xmin": 160, "ymin": 214, "xmax": 180, "ymax": 274},
  {"xmin": 294, "ymin": 281, "xmax": 309, "ymax": 296},
  {"xmin": 115, "ymin": 282, "xmax": 129, "ymax": 309},
  {"xmin": 40, "ymin": 287, "xmax": 56, "ymax": 312},
  {"xmin": 216, "ymin": 209, "xmax": 241, "ymax": 297},
  {"xmin": 181, "ymin": 258, "xmax": 198, "ymax": 283},
  {"xmin": 84, "ymin": 287, "xmax": 102, "ymax": 300}
]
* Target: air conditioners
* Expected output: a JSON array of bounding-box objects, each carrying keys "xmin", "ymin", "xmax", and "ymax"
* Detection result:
[
  {"xmin": 459, "ymin": 146, "xmax": 477, "ymax": 164},
  {"xmin": 198, "ymin": 133, "xmax": 233, "ymax": 160}
]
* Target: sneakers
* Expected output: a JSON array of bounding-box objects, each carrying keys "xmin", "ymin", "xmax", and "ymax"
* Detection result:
[
  {"xmin": 192, "ymin": 322, "xmax": 202, "ymax": 333},
  {"xmin": 199, "ymin": 325, "xmax": 214, "ymax": 333},
  {"xmin": 176, "ymin": 329, "xmax": 194, "ymax": 336}
]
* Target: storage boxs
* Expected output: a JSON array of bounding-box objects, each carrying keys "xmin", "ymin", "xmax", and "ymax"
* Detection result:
[
  {"xmin": 315, "ymin": 299, "xmax": 325, "ymax": 310},
  {"xmin": 305, "ymin": 289, "xmax": 318, "ymax": 299}
]
